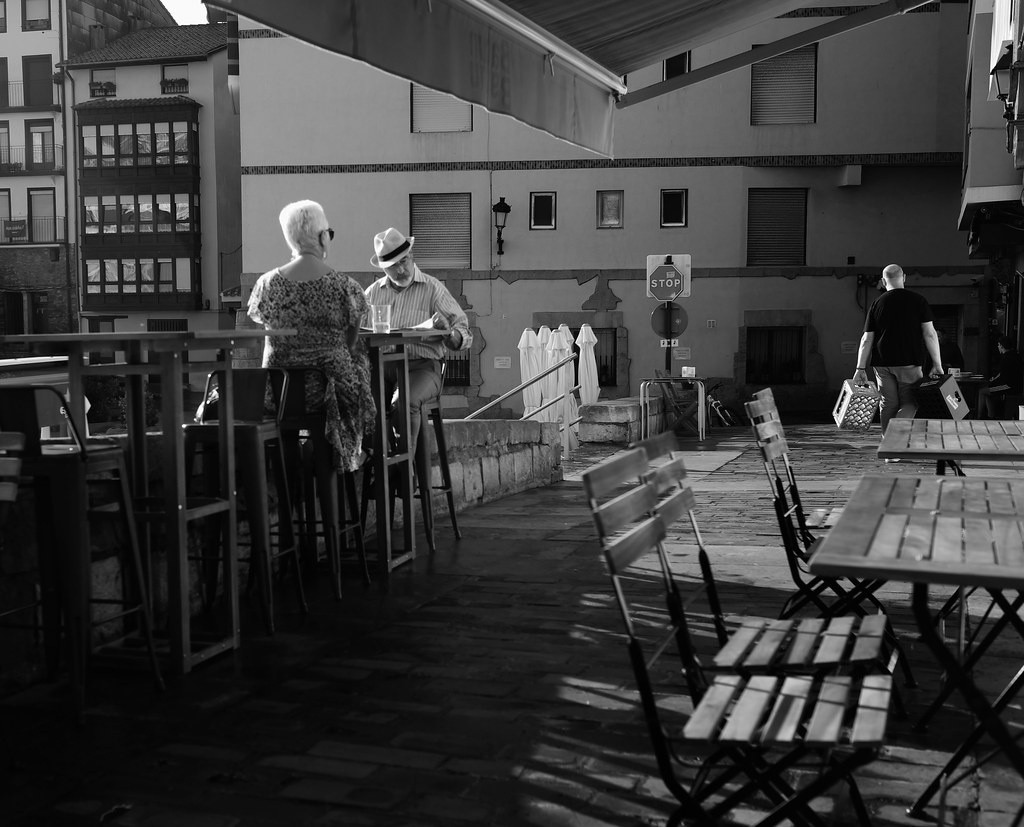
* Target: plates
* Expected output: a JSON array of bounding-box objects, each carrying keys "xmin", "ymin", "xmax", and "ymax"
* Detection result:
[{"xmin": 970, "ymin": 377, "xmax": 984, "ymax": 380}]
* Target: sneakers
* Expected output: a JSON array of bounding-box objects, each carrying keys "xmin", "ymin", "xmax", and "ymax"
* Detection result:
[
  {"xmin": 885, "ymin": 457, "xmax": 899, "ymax": 463},
  {"xmin": 398, "ymin": 459, "xmax": 418, "ymax": 500}
]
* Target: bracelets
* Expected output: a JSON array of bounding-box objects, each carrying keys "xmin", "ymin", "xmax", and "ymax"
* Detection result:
[
  {"xmin": 443, "ymin": 327, "xmax": 455, "ymax": 339},
  {"xmin": 856, "ymin": 367, "xmax": 866, "ymax": 371}
]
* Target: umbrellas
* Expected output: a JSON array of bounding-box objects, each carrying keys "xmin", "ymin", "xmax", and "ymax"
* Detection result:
[
  {"xmin": 65, "ymin": 383, "xmax": 91, "ymax": 440},
  {"xmin": 518, "ymin": 323, "xmax": 601, "ymax": 451}
]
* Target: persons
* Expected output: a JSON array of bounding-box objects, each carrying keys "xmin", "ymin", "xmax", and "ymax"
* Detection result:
[
  {"xmin": 923, "ymin": 327, "xmax": 964, "ymax": 373},
  {"xmin": 853, "ymin": 264, "xmax": 944, "ymax": 463},
  {"xmin": 976, "ymin": 335, "xmax": 1024, "ymax": 420},
  {"xmin": 363, "ymin": 227, "xmax": 473, "ymax": 499},
  {"xmin": 247, "ymin": 199, "xmax": 375, "ymax": 473}
]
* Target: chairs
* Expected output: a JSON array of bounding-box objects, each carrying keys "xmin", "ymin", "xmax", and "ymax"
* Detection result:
[
  {"xmin": 0, "ymin": 355, "xmax": 462, "ymax": 730},
  {"xmin": 655, "ymin": 368, "xmax": 712, "ymax": 436},
  {"xmin": 581, "ymin": 387, "xmax": 920, "ymax": 827}
]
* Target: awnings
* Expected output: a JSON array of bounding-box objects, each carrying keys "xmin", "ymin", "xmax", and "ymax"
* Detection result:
[{"xmin": 200, "ymin": 0, "xmax": 935, "ymax": 160}]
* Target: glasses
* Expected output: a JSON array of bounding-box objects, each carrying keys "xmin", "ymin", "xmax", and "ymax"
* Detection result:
[{"xmin": 323, "ymin": 227, "xmax": 334, "ymax": 240}]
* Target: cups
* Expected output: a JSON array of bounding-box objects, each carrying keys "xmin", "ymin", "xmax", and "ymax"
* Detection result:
[{"xmin": 371, "ymin": 304, "xmax": 391, "ymax": 334}]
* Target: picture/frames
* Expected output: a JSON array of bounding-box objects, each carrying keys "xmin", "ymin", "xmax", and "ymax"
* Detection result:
[
  {"xmin": 660, "ymin": 187, "xmax": 689, "ymax": 228},
  {"xmin": 528, "ymin": 190, "xmax": 558, "ymax": 231},
  {"xmin": 595, "ymin": 190, "xmax": 624, "ymax": 230}
]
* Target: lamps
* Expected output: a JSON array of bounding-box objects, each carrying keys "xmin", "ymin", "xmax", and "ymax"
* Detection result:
[
  {"xmin": 492, "ymin": 196, "xmax": 512, "ymax": 255},
  {"xmin": 989, "ymin": 42, "xmax": 1014, "ymax": 118}
]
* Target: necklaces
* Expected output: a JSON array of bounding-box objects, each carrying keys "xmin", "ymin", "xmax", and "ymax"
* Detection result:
[{"xmin": 296, "ymin": 252, "xmax": 320, "ymax": 260}]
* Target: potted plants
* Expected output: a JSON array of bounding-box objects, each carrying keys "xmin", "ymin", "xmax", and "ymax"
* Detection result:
[
  {"xmin": 89, "ymin": 81, "xmax": 103, "ymax": 90},
  {"xmin": 160, "ymin": 78, "xmax": 174, "ymax": 87},
  {"xmin": 102, "ymin": 82, "xmax": 115, "ymax": 93},
  {"xmin": 50, "ymin": 72, "xmax": 69, "ymax": 85},
  {"xmin": 176, "ymin": 78, "xmax": 188, "ymax": 87}
]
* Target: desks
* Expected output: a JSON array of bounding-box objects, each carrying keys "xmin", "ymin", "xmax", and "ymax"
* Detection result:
[
  {"xmin": 808, "ymin": 474, "xmax": 1024, "ymax": 827},
  {"xmin": 359, "ymin": 328, "xmax": 450, "ymax": 572},
  {"xmin": 955, "ymin": 376, "xmax": 989, "ymax": 420},
  {"xmin": 638, "ymin": 377, "xmax": 709, "ymax": 442},
  {"xmin": 876, "ymin": 418, "xmax": 1024, "ymax": 662},
  {"xmin": 0, "ymin": 327, "xmax": 299, "ymax": 674}
]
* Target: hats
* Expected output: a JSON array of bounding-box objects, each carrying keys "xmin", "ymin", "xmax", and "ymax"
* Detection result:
[{"xmin": 370, "ymin": 227, "xmax": 415, "ymax": 269}]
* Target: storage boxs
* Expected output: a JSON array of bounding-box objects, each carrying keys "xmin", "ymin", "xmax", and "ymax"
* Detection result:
[
  {"xmin": 832, "ymin": 378, "xmax": 879, "ymax": 432},
  {"xmin": 919, "ymin": 374, "xmax": 969, "ymax": 420}
]
